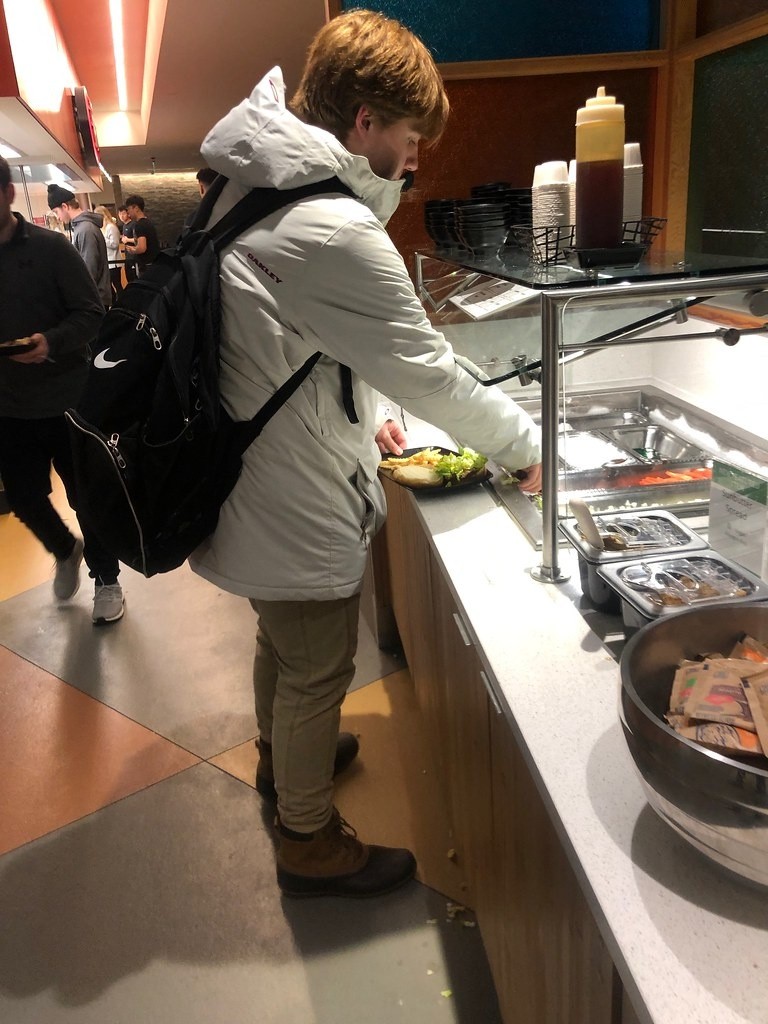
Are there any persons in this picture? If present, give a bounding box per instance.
[1,156,130,626]
[181,167,221,237]
[43,180,159,317]
[191,4,543,895]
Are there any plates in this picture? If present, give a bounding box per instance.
[369,446,494,497]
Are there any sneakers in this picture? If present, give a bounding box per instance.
[92,575,125,625]
[53,539,84,600]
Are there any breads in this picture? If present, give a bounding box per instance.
[392,465,444,488]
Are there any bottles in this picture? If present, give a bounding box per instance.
[575,86,625,248]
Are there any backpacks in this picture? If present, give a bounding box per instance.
[52,177,363,579]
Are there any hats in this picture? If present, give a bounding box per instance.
[47,184,75,210]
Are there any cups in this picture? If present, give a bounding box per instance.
[623,143,643,222]
[531,159,576,263]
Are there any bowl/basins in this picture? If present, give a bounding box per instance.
[424,182,531,256]
[617,602,768,886]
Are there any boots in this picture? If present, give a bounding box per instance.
[255,731,360,797]
[274,809,417,900]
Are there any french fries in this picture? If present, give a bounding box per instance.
[380,447,444,468]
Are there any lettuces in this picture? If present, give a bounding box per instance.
[435,446,489,487]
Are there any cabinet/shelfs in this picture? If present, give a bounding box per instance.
[379,472,639,1024]
